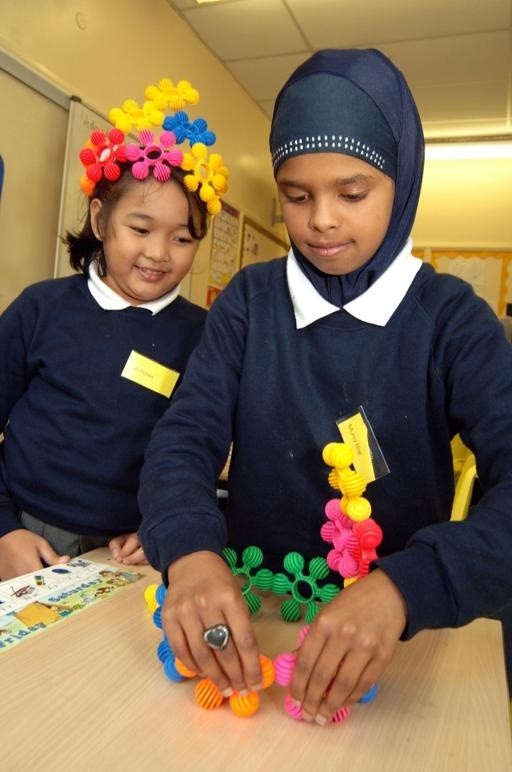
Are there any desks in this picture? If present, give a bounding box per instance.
[0,541,512,772]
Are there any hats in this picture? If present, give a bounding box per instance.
[271,73,397,179]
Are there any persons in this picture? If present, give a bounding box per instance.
[0,147,209,589]
[135,48,512,725]
